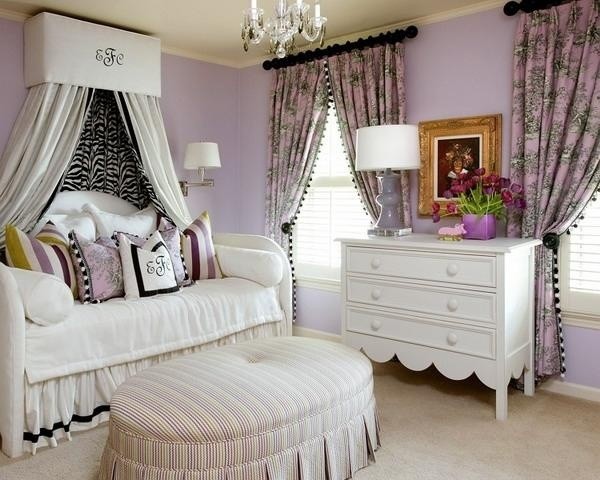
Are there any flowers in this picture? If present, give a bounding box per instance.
[430,163,530,226]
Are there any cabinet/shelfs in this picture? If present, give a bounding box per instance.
[338,235,542,424]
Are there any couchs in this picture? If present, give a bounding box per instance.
[1,189,293,461]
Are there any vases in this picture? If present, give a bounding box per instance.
[463,211,497,241]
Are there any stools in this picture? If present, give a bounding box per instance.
[97,334,382,480]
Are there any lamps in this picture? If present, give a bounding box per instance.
[238,1,329,60]
[174,136,222,198]
[354,123,423,237]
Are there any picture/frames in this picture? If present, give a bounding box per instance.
[419,113,504,219]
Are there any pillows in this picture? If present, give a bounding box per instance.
[210,242,285,287]
[1,265,77,326]
[3,205,226,303]
